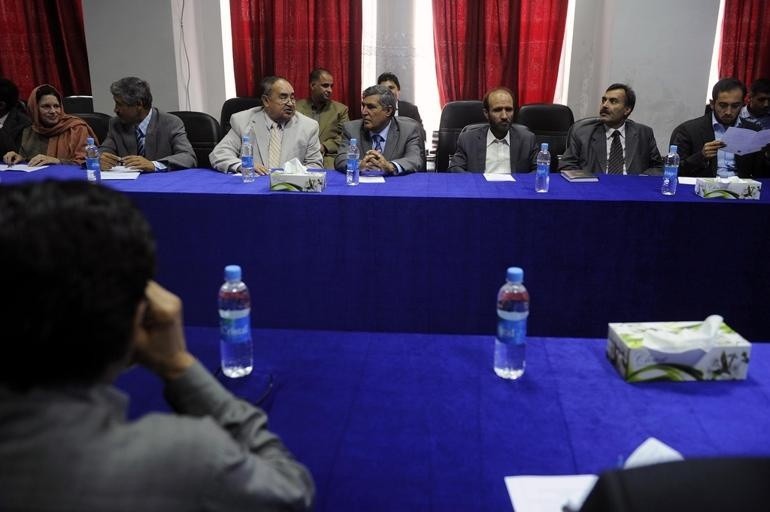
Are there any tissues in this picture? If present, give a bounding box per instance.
[606,314,753,384]
[694,175,762,201]
[269,156,326,192]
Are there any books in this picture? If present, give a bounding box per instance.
[561,170,599,183]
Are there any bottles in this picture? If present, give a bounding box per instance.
[492,267,530,380]
[535,143,551,193]
[346,138,360,186]
[86,138,101,185]
[661,145,681,196]
[217,264,253,378]
[241,136,255,183]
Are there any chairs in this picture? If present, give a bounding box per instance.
[166,112,220,168]
[19,95,111,145]
[435,101,489,172]
[220,97,264,140]
[516,104,574,173]
[565,117,634,150]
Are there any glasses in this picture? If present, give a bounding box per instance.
[269,97,295,105]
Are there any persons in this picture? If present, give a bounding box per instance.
[0,176,314,512]
[738,78,770,129]
[668,78,770,180]
[557,83,667,177]
[2,84,101,167]
[296,67,350,170]
[208,75,324,176]
[576,453,770,512]
[334,84,426,176]
[448,87,541,173]
[97,77,198,172]
[0,78,32,163]
[377,72,423,128]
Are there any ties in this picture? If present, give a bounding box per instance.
[373,135,382,150]
[135,126,144,156]
[268,124,282,168]
[608,130,623,175]
